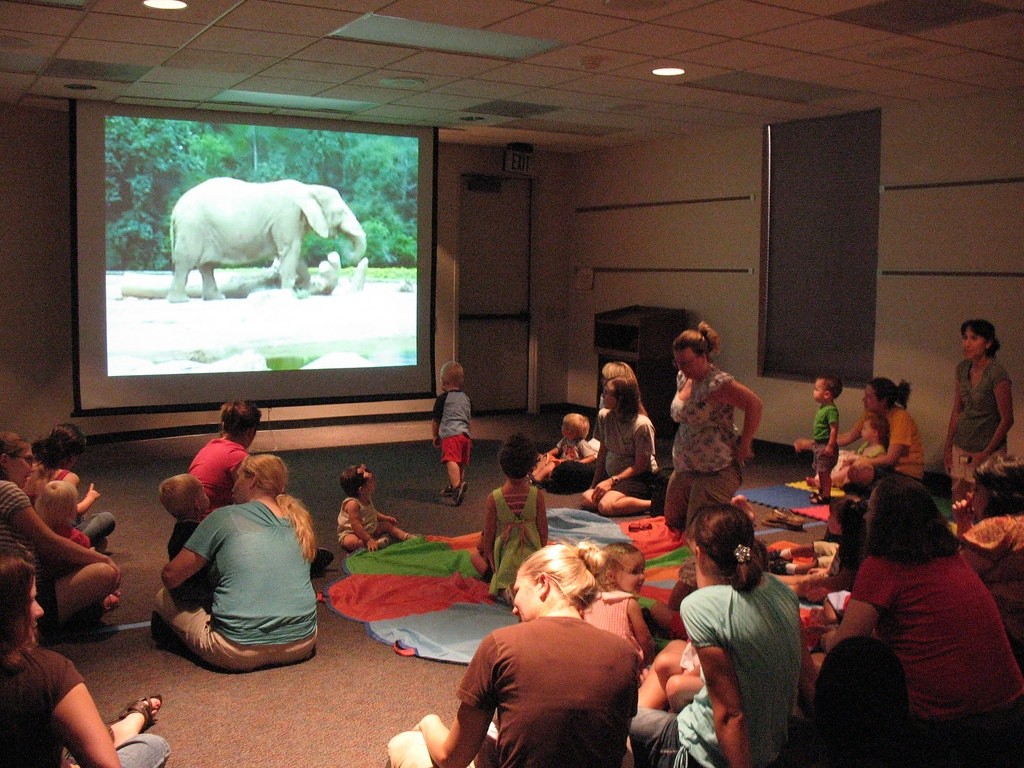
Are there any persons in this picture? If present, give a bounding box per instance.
[0,554,169,768]
[36,480,91,573]
[189,401,334,569]
[160,474,215,612]
[152,454,318,672]
[23,423,115,554]
[336,464,415,552]
[389,321,1024,768]
[0,432,121,639]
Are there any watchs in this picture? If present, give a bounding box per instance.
[612,475,619,485]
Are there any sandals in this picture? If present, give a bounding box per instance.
[440,484,454,497]
[104,693,162,741]
[454,481,468,506]
[809,490,831,506]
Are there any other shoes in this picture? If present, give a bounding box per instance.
[313,549,333,573]
[101,593,118,609]
[770,561,789,574]
[405,533,421,541]
[767,550,795,562]
[376,539,390,548]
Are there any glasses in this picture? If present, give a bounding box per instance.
[671,355,701,368]
[8,451,33,465]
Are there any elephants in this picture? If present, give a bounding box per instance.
[167,176,367,303]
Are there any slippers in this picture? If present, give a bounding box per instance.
[762,510,803,532]
